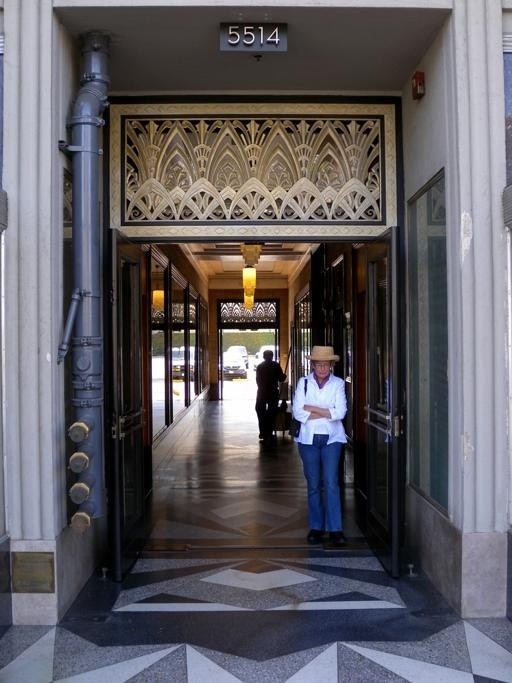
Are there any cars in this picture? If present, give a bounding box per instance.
[221,350,245,378]
[253,344,278,368]
[227,345,249,368]
[169,346,195,381]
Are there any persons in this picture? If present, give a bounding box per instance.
[289,344,352,548]
[255,349,287,442]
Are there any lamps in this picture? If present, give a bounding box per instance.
[151,263,164,311]
[239,263,257,310]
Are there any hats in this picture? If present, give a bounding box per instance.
[306,346,341,363]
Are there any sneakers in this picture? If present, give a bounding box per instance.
[306,529,345,549]
